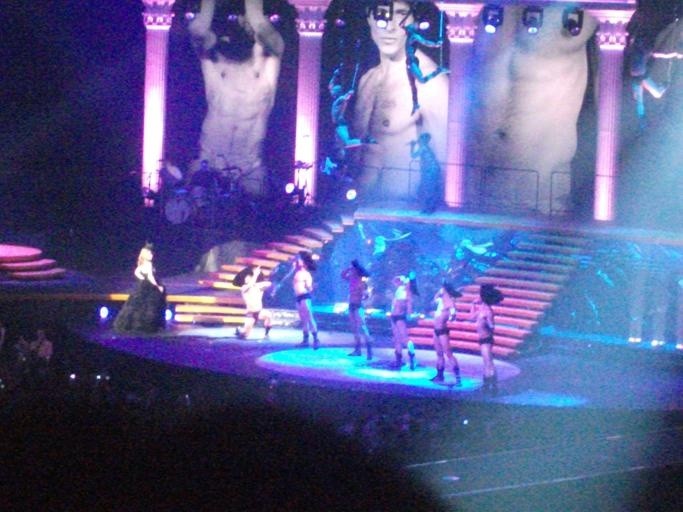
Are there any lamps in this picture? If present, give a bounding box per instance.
[481,3,504,35]
[407,0,433,30]
[370,1,395,29]
[562,5,585,37]
[521,3,543,36]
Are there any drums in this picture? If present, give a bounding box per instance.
[160,163,182,187]
[207,188,224,203]
[191,186,210,209]
[164,189,198,226]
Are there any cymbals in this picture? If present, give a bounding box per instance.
[229,172,236,174]
[223,166,236,171]
[223,176,231,179]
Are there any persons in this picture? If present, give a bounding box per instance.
[293,251,321,349]
[353,1,450,209]
[327,59,379,162]
[340,258,373,360]
[106,148,356,274]
[397,6,452,118]
[540,244,683,344]
[113,243,169,336]
[352,219,517,317]
[430,282,464,387]
[470,0,600,216]
[470,283,505,393]
[2,315,448,511]
[408,132,444,217]
[231,264,273,341]
[626,13,680,137]
[186,0,285,197]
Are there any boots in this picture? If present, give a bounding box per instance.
[453,365,461,387]
[234,326,241,336]
[312,332,319,349]
[348,344,361,356]
[296,331,308,347]
[489,372,497,396]
[432,364,444,382]
[408,350,415,369]
[263,326,271,340]
[477,374,489,392]
[388,351,401,366]
[367,343,373,359]
